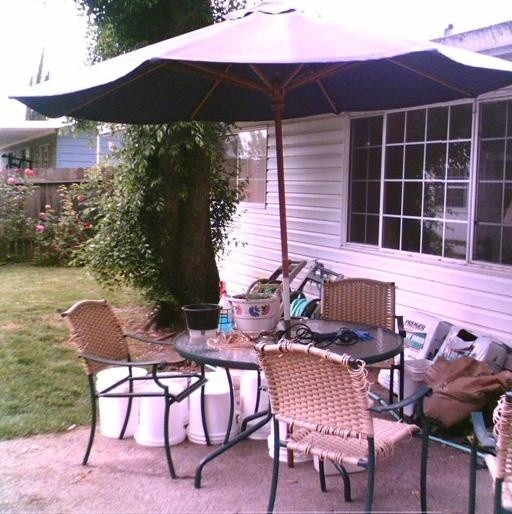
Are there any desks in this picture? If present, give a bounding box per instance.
[174,320,406,489]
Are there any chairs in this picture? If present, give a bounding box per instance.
[255,278,512,513]
[62,297,210,480]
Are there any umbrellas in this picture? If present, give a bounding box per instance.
[7,2,512,341]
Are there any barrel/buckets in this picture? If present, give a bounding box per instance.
[230,293,277,333]
[93,367,366,476]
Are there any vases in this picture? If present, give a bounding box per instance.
[180,304,224,341]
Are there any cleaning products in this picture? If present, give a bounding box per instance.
[217,293,235,332]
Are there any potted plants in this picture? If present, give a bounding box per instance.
[230,284,280,338]
